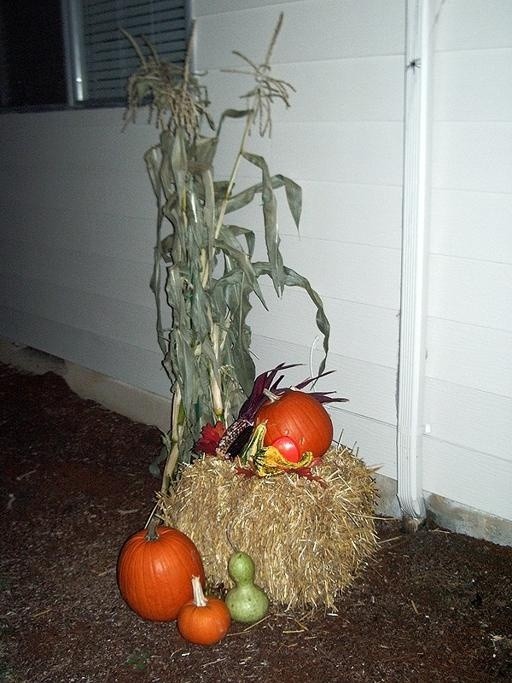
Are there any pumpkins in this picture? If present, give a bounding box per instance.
[117,516,206,623]
[240,388,333,478]
[178,575,229,645]
